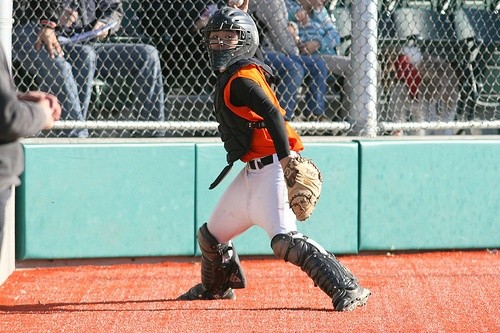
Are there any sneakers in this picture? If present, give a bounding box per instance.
[175,284,236,300]
[336,288,372,311]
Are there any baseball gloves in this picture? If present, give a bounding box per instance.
[282,156,324,221]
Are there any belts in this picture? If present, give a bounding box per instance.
[247,155,276,170]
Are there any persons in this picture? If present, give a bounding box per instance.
[174,7,372,314]
[194,0,341,123]
[12,0,166,139]
[0,42,65,290]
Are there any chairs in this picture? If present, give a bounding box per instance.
[327,0,500,107]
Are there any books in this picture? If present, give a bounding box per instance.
[59,20,118,46]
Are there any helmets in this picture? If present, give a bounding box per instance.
[200,7,260,72]
